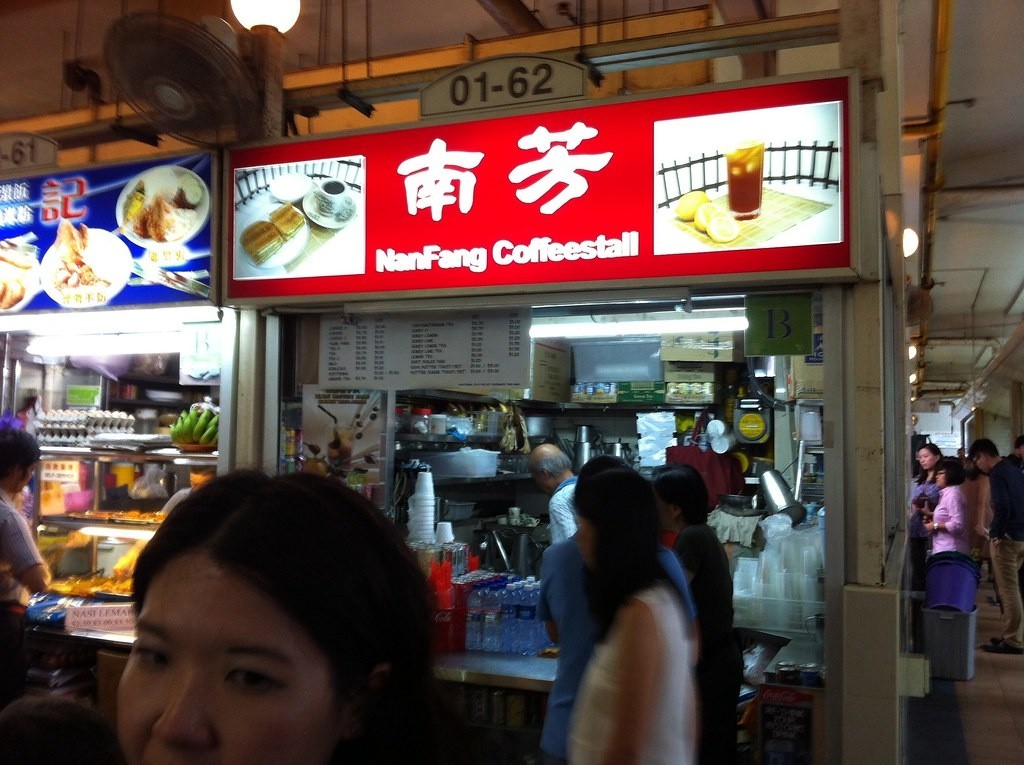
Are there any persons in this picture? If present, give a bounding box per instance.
[969,438,1024,654]
[117,469,433,765]
[529,444,581,544]
[0,427,53,713]
[537,454,632,765]
[652,464,743,765]
[923,459,968,555]
[567,466,696,765]
[907,443,943,653]
[957,435,1024,616]
[160,465,217,514]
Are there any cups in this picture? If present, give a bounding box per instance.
[436,522,454,544]
[510,518,521,526]
[727,140,764,221]
[509,507,521,518]
[804,613,825,643]
[414,472,435,542]
[327,424,354,478]
[733,532,824,613]
[497,517,507,525]
[317,179,354,217]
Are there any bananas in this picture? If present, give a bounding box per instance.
[169,409,219,447]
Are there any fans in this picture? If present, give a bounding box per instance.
[907,275,933,327]
[104,12,264,149]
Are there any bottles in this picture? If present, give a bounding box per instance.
[464,576,556,654]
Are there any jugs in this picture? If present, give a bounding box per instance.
[522,516,540,527]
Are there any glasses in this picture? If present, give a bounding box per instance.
[973,455,981,465]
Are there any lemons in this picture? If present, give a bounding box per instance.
[675,190,740,242]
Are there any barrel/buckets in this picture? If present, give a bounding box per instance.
[923,551,982,613]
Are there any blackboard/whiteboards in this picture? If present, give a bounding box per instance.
[756,700,828,765]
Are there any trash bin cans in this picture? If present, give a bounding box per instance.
[919,550,983,681]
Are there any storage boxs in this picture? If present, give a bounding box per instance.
[789,324,823,398]
[410,449,501,478]
[97,647,131,735]
[443,501,476,521]
[495,330,744,406]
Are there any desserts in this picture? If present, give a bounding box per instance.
[239,202,305,266]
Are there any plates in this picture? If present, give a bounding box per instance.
[240,203,309,269]
[41,229,132,308]
[146,390,184,402]
[303,190,356,229]
[115,165,210,249]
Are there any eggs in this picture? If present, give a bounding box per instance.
[44,409,127,428]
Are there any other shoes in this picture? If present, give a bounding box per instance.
[984,637,1024,655]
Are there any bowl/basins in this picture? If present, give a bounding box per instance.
[718,494,760,517]
[271,173,311,205]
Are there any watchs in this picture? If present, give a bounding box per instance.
[934,522,939,532]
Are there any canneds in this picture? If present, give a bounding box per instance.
[390,542,501,611]
[572,382,618,395]
[667,382,714,395]
[393,407,447,435]
[280,427,304,475]
[774,661,822,689]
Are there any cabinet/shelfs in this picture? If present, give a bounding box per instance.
[60,372,195,412]
[32,447,218,600]
[369,390,547,531]
